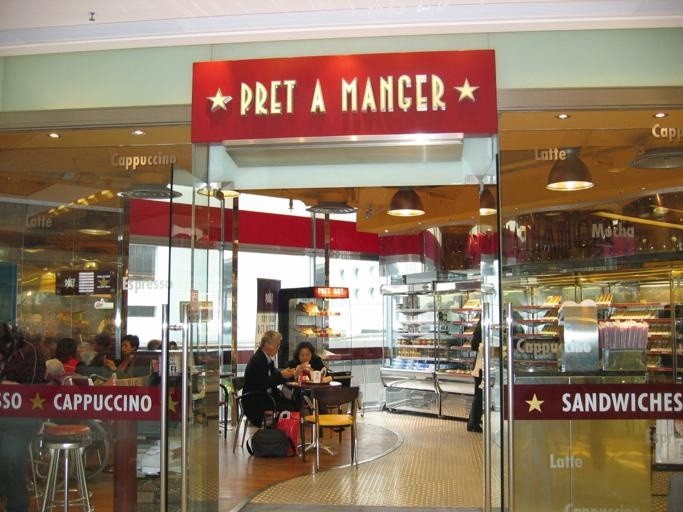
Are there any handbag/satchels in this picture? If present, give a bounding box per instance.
[246,428,296,457]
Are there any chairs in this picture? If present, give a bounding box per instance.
[26,370,358,510]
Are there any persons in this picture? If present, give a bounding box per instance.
[147,340,177,349]
[45,335,139,383]
[0,321,46,512]
[467,313,483,432]
[148,359,160,386]
[241,331,326,429]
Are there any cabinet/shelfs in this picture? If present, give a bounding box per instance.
[276,286,349,370]
[379,250,683,423]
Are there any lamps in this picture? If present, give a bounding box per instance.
[544,149,594,191]
[479,184,496,215]
[385,188,425,218]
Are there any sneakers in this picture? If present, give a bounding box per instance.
[467,423,482,432]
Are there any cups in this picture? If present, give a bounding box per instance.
[311,370,322,383]
